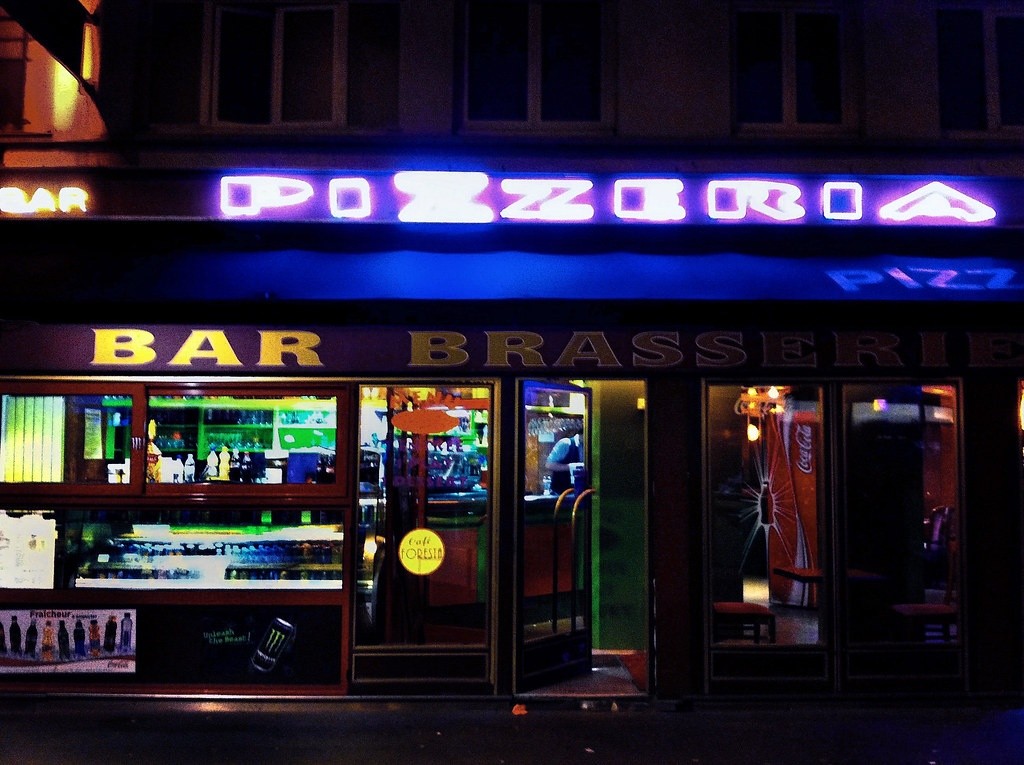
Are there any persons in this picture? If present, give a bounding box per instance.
[545,429,585,496]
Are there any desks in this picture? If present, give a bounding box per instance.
[773,567,880,646]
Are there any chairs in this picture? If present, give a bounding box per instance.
[714,602,777,643]
[892,541,960,645]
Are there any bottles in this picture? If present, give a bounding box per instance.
[185,454,195,483]
[120,613,132,652]
[207,446,218,476]
[172,454,184,484]
[73,621,86,656]
[241,451,254,484]
[58,620,70,659]
[88,620,101,656]
[42,621,54,661]
[9,616,22,656]
[761,480,774,525]
[104,616,117,652]
[230,449,241,482]
[108,543,342,580]
[219,447,231,479]
[24,619,38,658]
[0,622,7,654]
